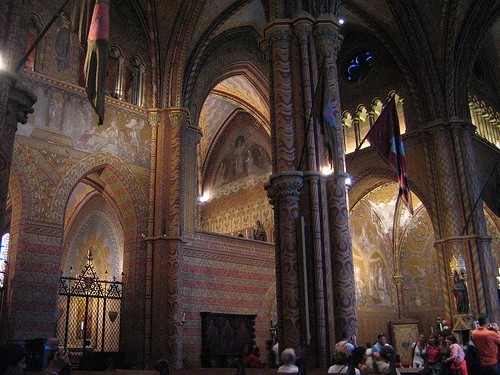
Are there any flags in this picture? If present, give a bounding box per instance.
[310,55,342,187]
[480,158,500,219]
[83,0,110,126]
[367,94,415,215]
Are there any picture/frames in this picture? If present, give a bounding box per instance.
[388,317,422,362]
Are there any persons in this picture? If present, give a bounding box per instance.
[203,315,252,357]
[411,312,500,375]
[252,221,266,241]
[469,313,500,375]
[0,337,71,375]
[271,330,398,374]
[452,269,469,313]
